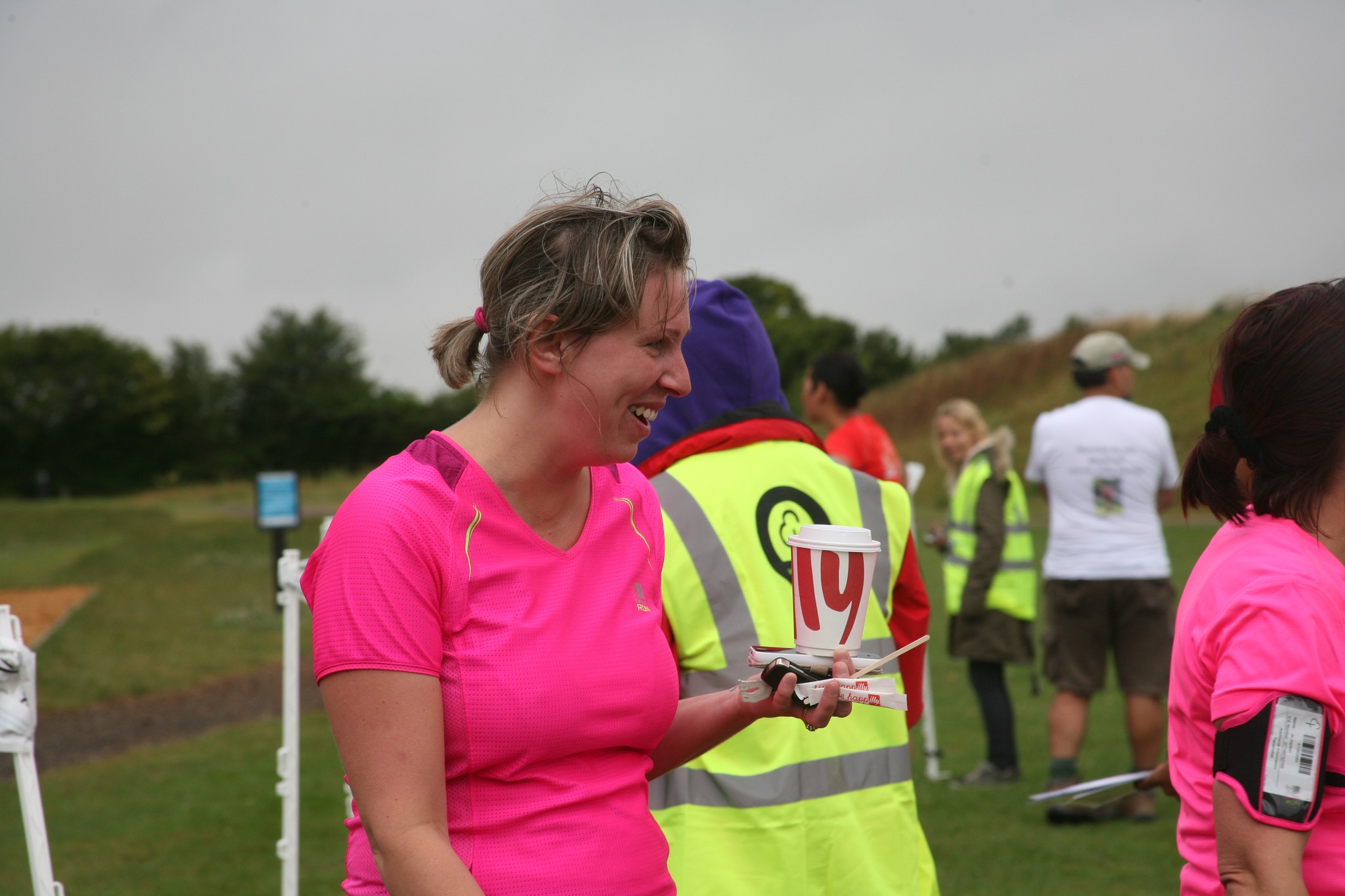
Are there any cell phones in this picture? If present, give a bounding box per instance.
[749,645,885,670]
[1260,695,1325,823]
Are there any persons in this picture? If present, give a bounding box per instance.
[801,350,909,488]
[1167,277,1345,896]
[927,398,1038,790]
[1027,330,1181,819]
[301,173,855,896]
[630,280,942,896]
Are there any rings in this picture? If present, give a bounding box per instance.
[803,708,818,731]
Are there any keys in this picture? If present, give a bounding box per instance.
[761,656,825,706]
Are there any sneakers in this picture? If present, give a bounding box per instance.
[949,761,1019,787]
[1042,774,1158,821]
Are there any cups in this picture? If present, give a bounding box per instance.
[787,525,882,656]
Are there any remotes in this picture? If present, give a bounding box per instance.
[760,657,825,702]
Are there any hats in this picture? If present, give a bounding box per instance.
[1069,332,1151,371]
